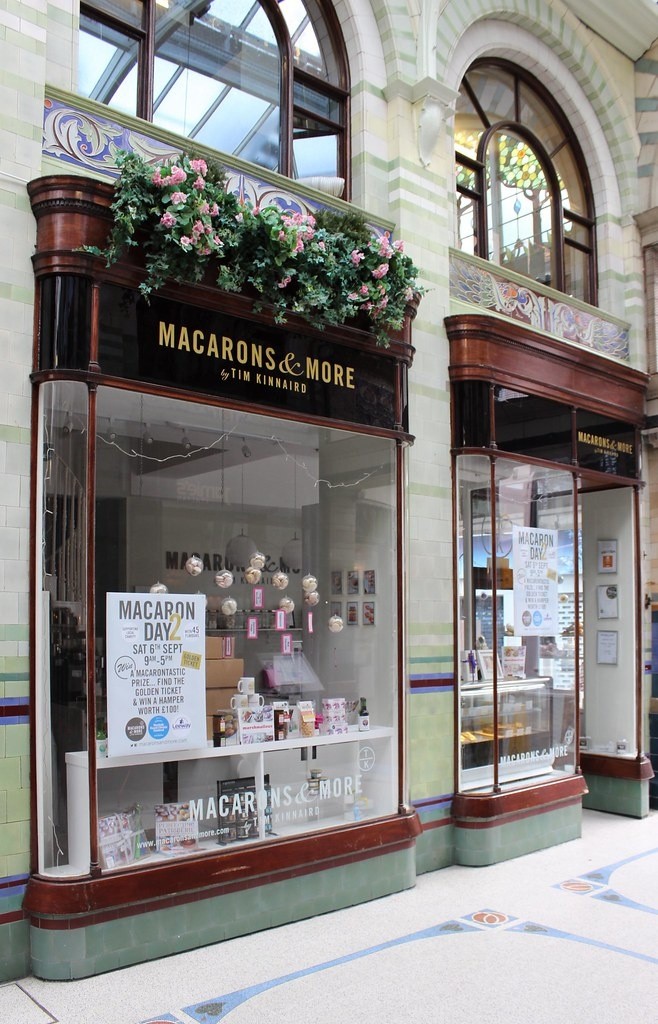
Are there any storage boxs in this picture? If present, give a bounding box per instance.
[205,634,245,740]
[220,706,275,747]
[97,808,150,869]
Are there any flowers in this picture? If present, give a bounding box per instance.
[69,146,432,350]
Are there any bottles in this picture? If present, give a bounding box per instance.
[358,698,370,731]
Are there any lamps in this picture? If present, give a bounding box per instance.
[141,423,155,445]
[237,438,252,458]
[181,430,193,449]
[61,411,74,433]
[107,417,117,441]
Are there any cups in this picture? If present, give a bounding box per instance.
[230,676,265,709]
